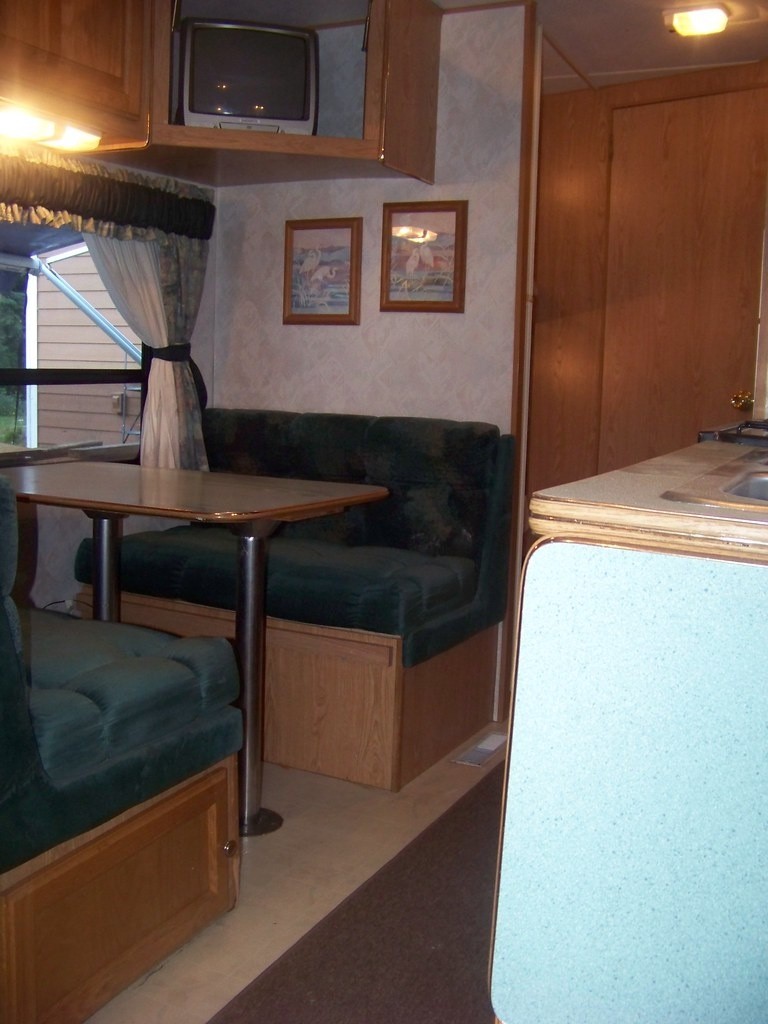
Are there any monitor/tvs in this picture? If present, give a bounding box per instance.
[167,17,321,136]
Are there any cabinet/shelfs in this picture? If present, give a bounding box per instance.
[0,0,441,189]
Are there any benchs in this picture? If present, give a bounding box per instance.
[0,473,242,1024]
[73,408,511,793]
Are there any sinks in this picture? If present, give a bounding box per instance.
[660,449,768,513]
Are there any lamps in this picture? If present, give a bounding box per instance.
[661,1,730,38]
[1,107,105,155]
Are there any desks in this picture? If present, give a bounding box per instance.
[1,460,389,836]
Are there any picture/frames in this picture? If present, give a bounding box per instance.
[282,217,362,324]
[379,199,468,314]
